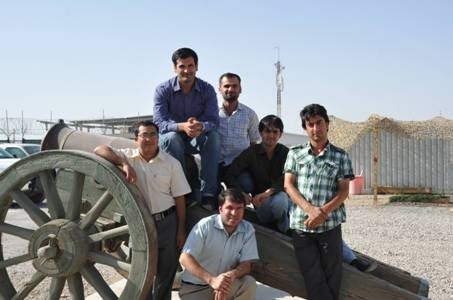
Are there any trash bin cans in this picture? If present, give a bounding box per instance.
[349,175,364,195]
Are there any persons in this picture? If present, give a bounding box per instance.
[95,121,191,300]
[178,187,261,300]
[152,48,221,213]
[224,115,378,273]
[218,73,260,194]
[283,104,355,300]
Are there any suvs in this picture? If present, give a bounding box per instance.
[2,141,40,160]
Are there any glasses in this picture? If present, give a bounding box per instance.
[138,133,158,140]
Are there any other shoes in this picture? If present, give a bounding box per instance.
[186,197,198,209]
[353,258,377,272]
[200,197,218,212]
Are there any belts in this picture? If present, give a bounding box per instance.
[181,280,209,286]
[150,206,176,221]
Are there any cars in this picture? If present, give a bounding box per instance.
[0,144,21,175]
